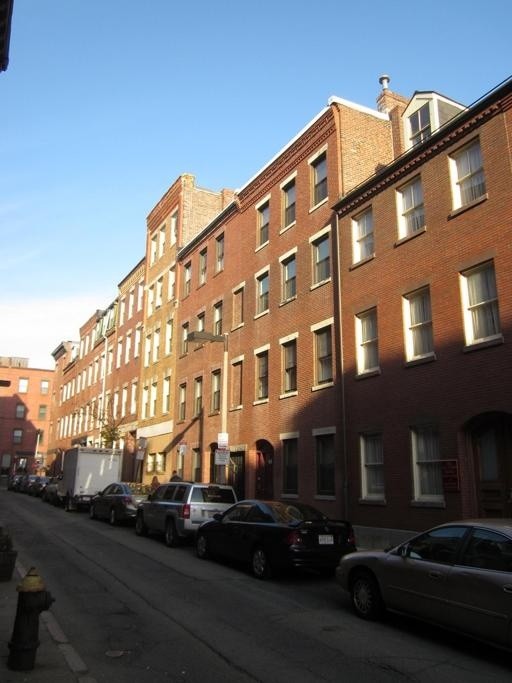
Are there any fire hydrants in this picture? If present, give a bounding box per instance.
[8,566,56,671]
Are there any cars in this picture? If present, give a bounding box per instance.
[330,518,512,657]
[7,472,59,506]
[194,499,357,579]
[88,481,157,526]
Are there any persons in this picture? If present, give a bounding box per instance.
[169,470,182,482]
[152,476,159,492]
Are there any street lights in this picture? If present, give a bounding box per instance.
[183,330,228,484]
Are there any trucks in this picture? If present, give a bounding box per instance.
[57,445,124,512]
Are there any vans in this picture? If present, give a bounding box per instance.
[135,480,238,547]
